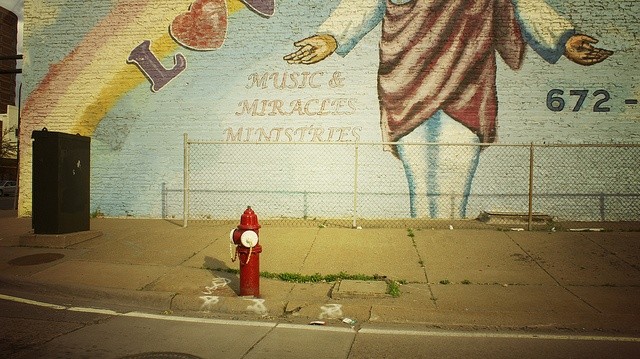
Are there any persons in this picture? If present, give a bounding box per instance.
[282,0,616,222]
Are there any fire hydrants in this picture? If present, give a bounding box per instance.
[228,206,263,297]
